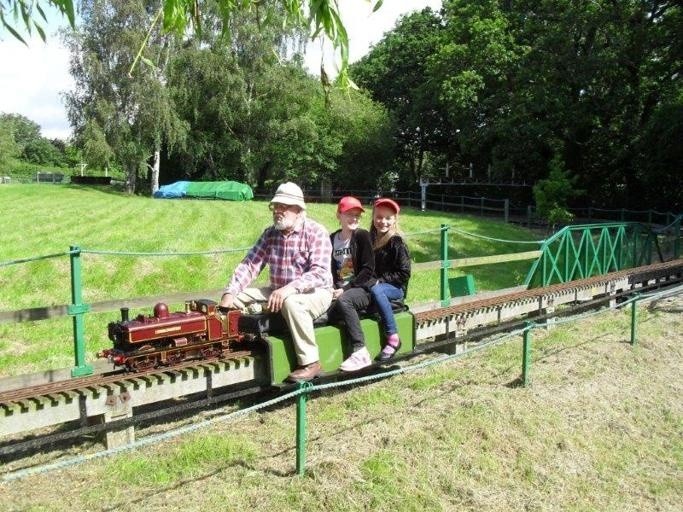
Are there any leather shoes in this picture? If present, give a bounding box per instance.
[378,338,401,360]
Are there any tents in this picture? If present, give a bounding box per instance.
[153,180,255,202]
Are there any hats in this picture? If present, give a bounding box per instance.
[338,197,365,214]
[374,198,400,214]
[269,181,308,210]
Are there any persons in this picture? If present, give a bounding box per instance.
[368,198,411,362]
[220,182,334,383]
[329,196,376,372]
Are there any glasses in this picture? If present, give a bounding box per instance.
[268,203,295,213]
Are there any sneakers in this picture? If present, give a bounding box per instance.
[287,362,321,382]
[338,351,373,372]
